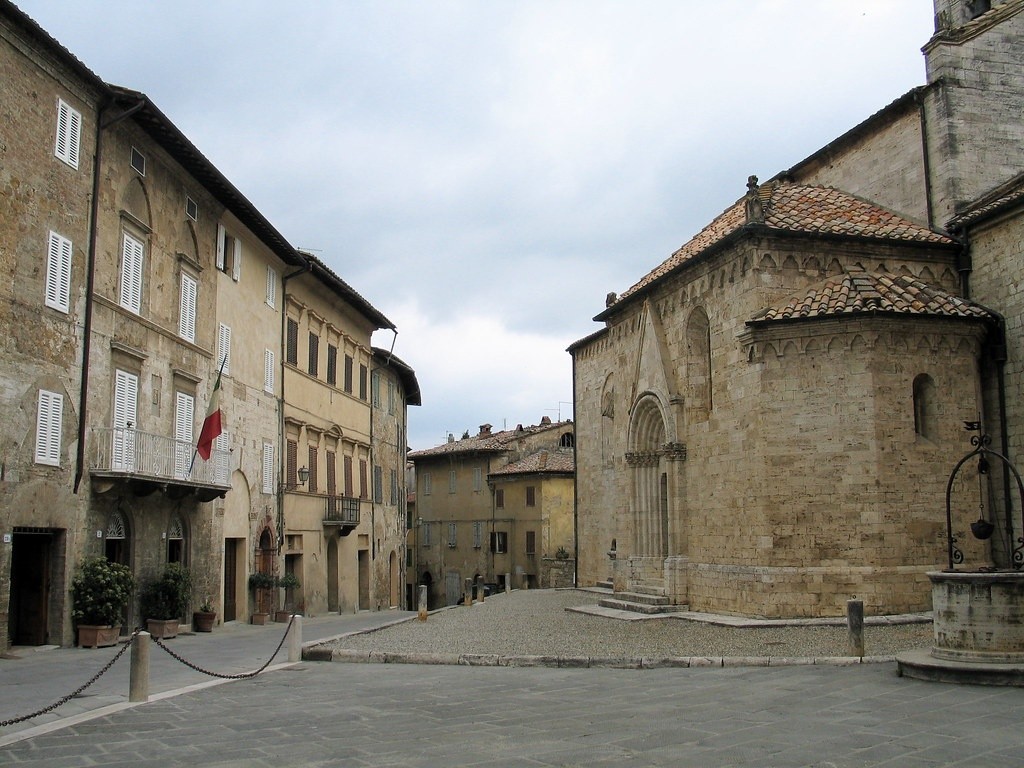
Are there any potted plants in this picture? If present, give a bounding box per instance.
[249,572,279,626]
[142,561,194,638]
[193,599,216,632]
[68,554,139,650]
[275,572,302,623]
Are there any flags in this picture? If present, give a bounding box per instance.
[197,370,223,462]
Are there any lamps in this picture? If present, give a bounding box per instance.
[276,465,310,500]
